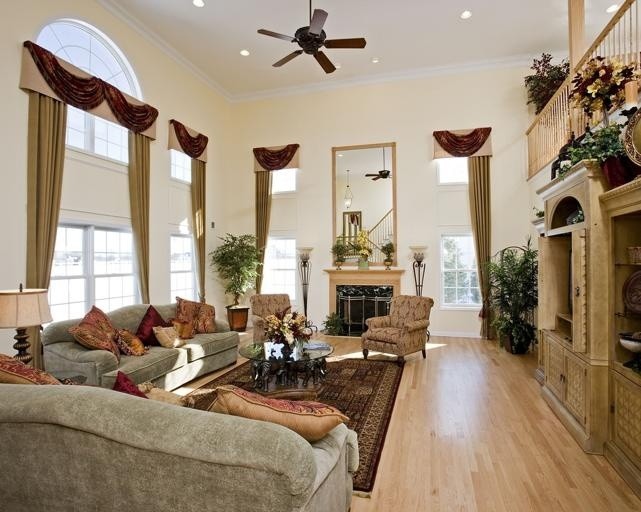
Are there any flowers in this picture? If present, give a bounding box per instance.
[263,307,320,343]
[566,56,637,155]
[356,229,373,258]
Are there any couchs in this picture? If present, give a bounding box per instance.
[40,302,241,394]
[0,383,360,512]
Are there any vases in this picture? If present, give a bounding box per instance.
[280,339,294,362]
[356,257,369,272]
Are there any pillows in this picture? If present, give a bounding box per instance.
[68,296,215,356]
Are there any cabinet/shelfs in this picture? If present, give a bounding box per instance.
[323,268,406,339]
[533,158,641,510]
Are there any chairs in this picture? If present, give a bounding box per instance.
[358,294,436,367]
[249,292,293,344]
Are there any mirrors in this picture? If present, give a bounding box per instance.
[332,141,396,267]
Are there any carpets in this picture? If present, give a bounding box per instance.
[177,353,407,493]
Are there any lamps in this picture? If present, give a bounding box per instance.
[0,281,54,367]
[295,246,318,334]
[343,170,353,210]
[408,245,430,342]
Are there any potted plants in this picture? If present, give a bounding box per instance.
[379,241,395,271]
[481,234,539,355]
[207,230,269,332]
[329,238,351,270]
[590,121,637,189]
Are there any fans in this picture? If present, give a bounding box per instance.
[364,147,393,181]
[255,1,368,75]
